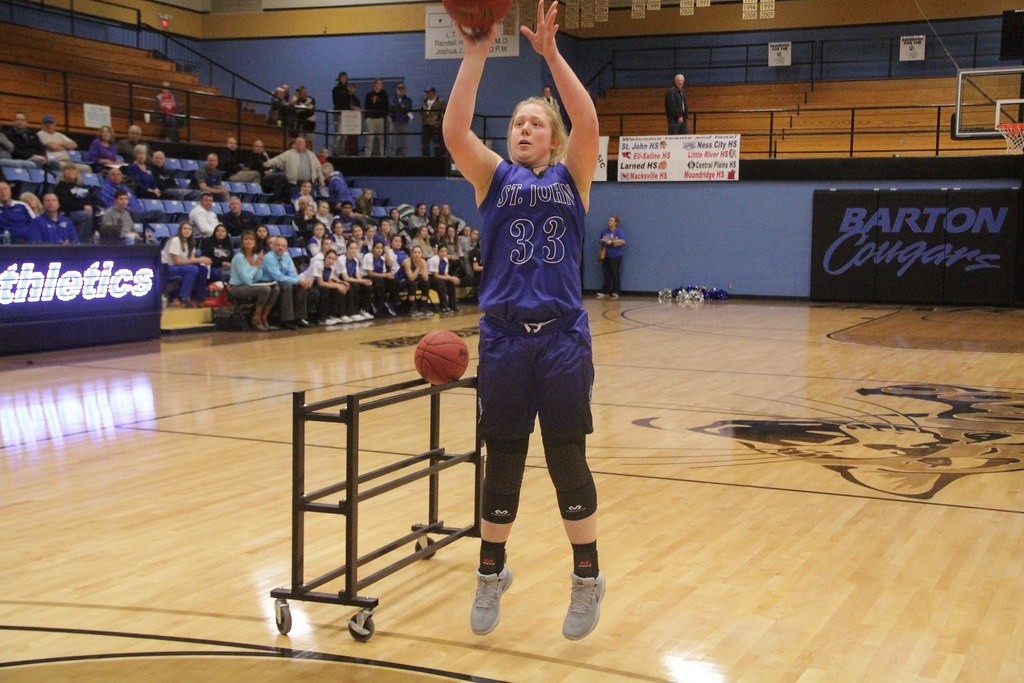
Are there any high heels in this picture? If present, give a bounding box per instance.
[263,320,276,330]
[252,319,268,331]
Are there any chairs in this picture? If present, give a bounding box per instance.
[0,148,450,332]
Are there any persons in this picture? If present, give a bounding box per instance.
[543,86,559,112]
[1,70,484,333]
[442,0,605,640]
[664,73,689,135]
[596,214,626,297]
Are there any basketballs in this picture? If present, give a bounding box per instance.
[413,328,470,386]
[440,0,515,28]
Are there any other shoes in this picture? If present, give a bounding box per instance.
[597,291,610,296]
[612,291,618,296]
[283,302,463,330]
[177,297,192,308]
[193,298,203,306]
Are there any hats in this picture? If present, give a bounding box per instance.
[425,86,436,93]
[43,116,55,124]
[398,84,405,89]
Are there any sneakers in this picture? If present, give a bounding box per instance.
[562,573,606,640]
[470,564,513,635]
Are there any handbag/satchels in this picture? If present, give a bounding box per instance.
[597,246,606,262]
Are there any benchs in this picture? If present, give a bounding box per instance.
[594,73,1024,159]
[0,20,316,151]
